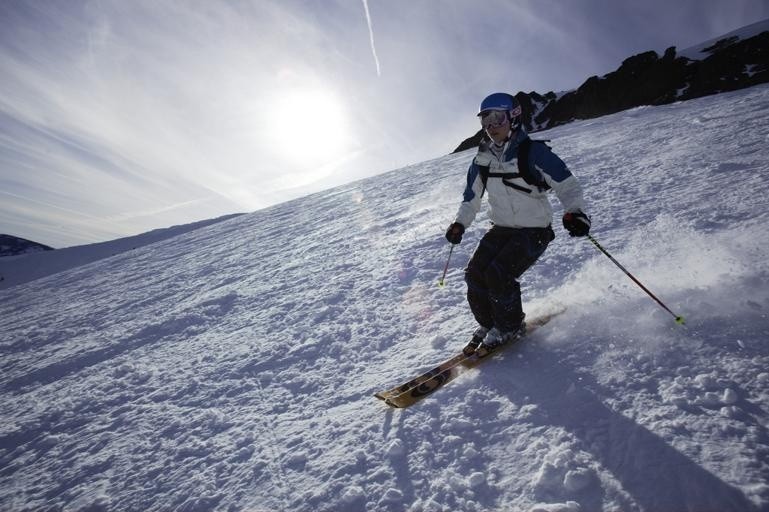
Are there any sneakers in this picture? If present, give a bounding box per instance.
[471,319,527,348]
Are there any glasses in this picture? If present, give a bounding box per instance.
[482,111,508,129]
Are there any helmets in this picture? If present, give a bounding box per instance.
[477,92,523,125]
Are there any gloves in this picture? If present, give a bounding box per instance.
[561,210,593,238]
[445,221,466,245]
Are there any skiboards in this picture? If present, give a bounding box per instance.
[374,309,565,408]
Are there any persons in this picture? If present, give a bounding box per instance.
[445,93,590,358]
